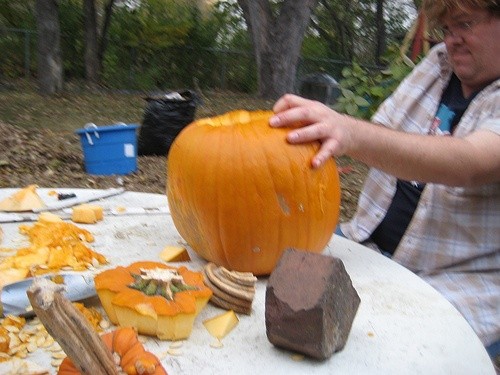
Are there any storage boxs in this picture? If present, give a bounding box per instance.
[73,123,142,175]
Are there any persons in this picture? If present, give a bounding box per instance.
[269,0,500,347]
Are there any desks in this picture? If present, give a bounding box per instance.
[0,187,496,375]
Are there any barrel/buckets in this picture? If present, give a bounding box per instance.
[75,122,141,176]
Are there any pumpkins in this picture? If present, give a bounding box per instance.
[165,109,342,277]
[26,278,169,375]
[94,260,214,341]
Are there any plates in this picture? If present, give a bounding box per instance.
[1,272,97,316]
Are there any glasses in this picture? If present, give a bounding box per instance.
[435,11,494,42]
